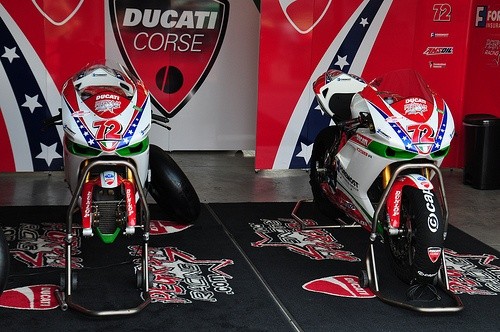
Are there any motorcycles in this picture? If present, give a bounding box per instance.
[46,63,172,275]
[309,68,458,288]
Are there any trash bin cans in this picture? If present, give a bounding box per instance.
[462,113,500,190]
